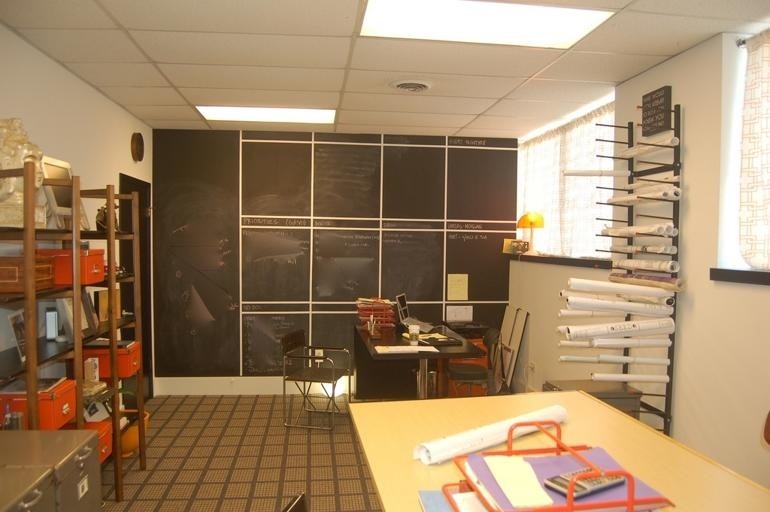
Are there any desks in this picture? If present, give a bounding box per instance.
[347,389,770,512]
[353,325,488,400]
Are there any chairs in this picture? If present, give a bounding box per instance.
[444,324,499,396]
[282,491,307,512]
[281,328,351,431]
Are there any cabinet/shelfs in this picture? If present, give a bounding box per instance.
[543,379,642,420]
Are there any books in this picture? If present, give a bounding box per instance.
[416,447,669,512]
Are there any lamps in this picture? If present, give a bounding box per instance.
[518,212,545,256]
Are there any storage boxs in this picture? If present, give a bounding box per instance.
[0,161,143,512]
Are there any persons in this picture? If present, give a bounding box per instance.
[0,120,51,229]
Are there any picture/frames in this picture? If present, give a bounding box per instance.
[511,241,529,257]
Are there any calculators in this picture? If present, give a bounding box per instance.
[544,468,625,498]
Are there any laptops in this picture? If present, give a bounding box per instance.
[396,292,434,333]
